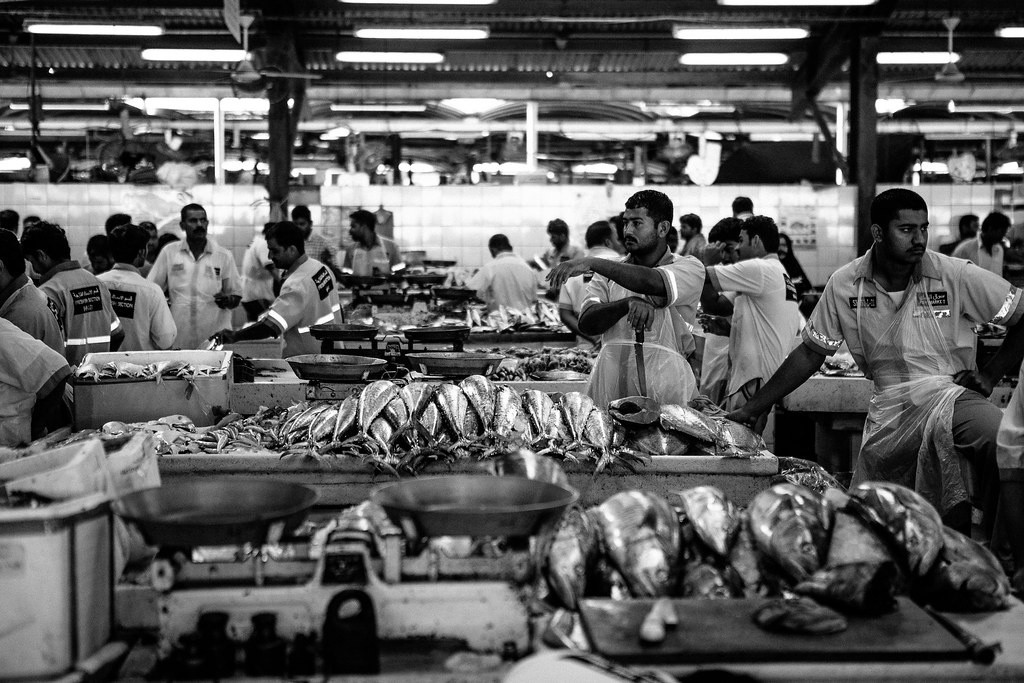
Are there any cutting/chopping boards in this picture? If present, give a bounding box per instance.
[575,594,969,663]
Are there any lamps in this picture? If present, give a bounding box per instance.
[671,23,812,41]
[352,24,491,41]
[23,19,165,37]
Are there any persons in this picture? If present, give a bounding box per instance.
[461,188,1024,583]
[0,203,406,447]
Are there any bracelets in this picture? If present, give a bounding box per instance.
[232,330,238,342]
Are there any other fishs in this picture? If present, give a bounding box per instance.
[821,354,867,378]
[403,266,480,289]
[545,479,1011,608]
[347,300,573,334]
[58,345,769,477]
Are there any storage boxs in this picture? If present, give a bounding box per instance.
[72,350,235,430]
[0,438,107,506]
[0,506,114,682]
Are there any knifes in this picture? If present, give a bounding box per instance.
[634,326,647,396]
